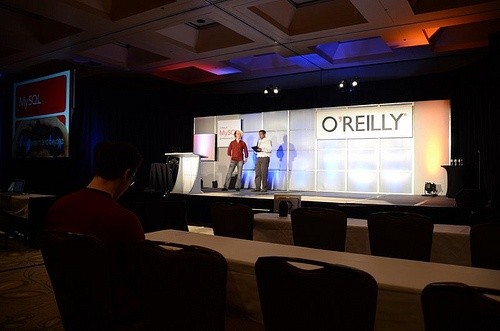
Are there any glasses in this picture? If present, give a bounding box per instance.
[121,169,137,185]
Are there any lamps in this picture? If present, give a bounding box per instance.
[351,75,360,88]
[338,79,347,88]
[274,83,280,94]
[264,84,273,94]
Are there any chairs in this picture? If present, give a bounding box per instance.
[255,257,378,331]
[211,202,255,240]
[469,224,500,270]
[146,201,189,234]
[421,283,500,331]
[367,211,434,262]
[292,207,347,251]
[118,241,228,331]
[37,231,122,331]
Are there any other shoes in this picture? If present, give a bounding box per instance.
[222,188,227,191]
[260,189,267,192]
[235,188,240,192]
[251,188,261,192]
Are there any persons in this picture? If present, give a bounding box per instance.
[45,138,144,243]
[220,130,248,191]
[252,130,272,192]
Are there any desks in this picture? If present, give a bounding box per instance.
[253,212,472,267]
[149,163,179,190]
[0,192,56,240]
[144,228,500,331]
